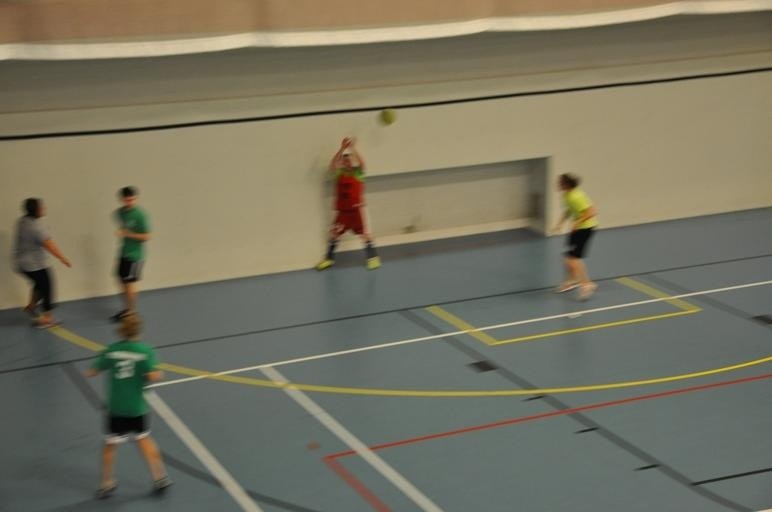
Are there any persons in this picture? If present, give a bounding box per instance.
[111,187,154,321]
[86,312,173,500]
[316,137,381,269]
[546,173,598,300]
[15,198,72,324]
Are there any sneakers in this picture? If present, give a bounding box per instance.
[154,477,174,492]
[317,260,335,270]
[367,256,381,270]
[108,308,137,324]
[24,304,63,329]
[553,280,597,301]
[94,483,118,500]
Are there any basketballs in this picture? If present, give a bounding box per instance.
[379,110,396,125]
